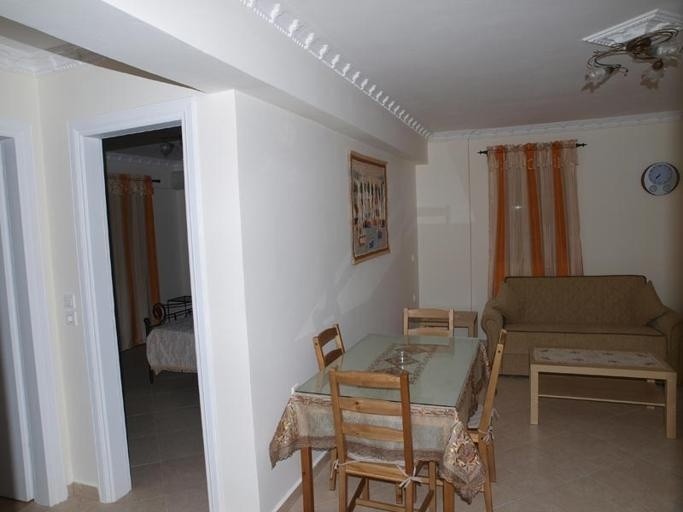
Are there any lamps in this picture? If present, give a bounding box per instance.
[578,9,681,98]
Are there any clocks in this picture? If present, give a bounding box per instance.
[641,161,680,198]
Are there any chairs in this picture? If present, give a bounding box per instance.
[464,330,507,512]
[312,323,348,492]
[328,366,436,511]
[403,307,454,337]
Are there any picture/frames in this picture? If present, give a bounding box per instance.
[349,149,391,265]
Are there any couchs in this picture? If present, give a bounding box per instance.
[480,275,682,385]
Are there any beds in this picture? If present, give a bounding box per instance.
[145,303,198,385]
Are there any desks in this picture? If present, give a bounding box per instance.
[528,346,677,440]
[267,332,500,511]
[418,311,478,338]
